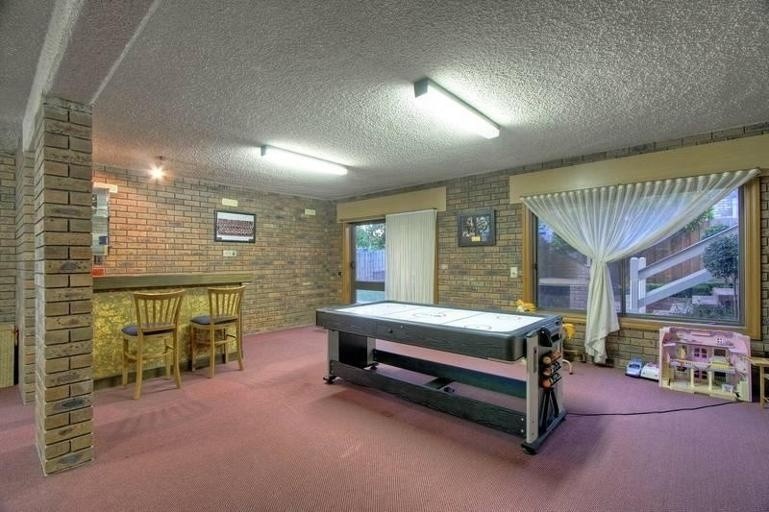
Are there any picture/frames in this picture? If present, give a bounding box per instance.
[214,210,256,244]
[457,209,496,246]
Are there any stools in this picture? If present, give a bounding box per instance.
[751,357,769,408]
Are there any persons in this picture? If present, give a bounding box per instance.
[217,218,254,235]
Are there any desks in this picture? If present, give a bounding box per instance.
[316,300,576,446]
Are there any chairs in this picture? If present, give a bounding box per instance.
[190,285,246,378]
[121,289,188,398]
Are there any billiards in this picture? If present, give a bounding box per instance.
[542,351,562,387]
